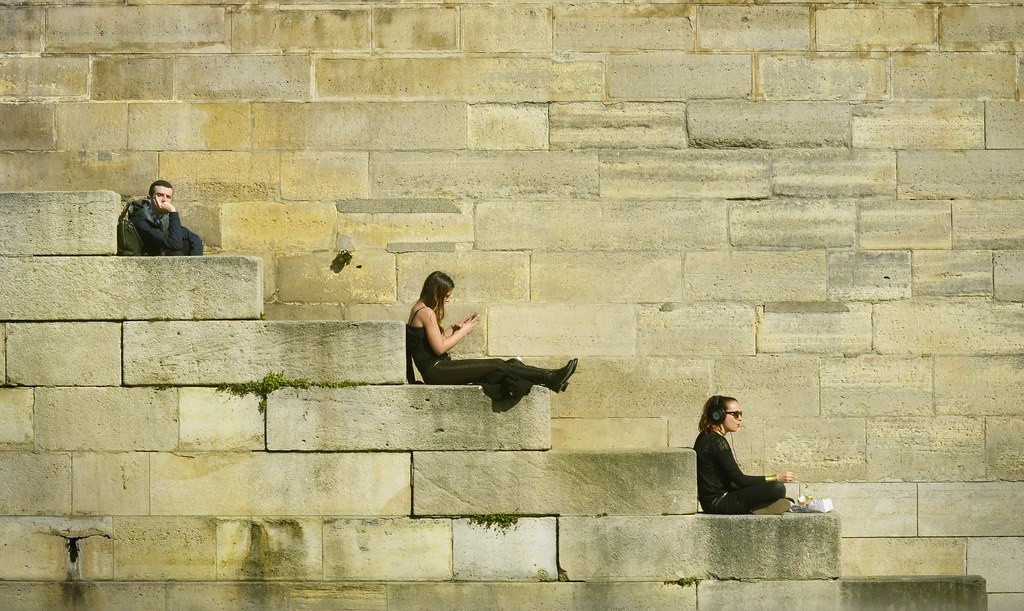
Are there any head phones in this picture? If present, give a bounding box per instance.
[709,395,726,424]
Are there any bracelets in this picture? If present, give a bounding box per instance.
[776,474,780,482]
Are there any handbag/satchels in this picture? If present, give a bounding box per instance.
[117,202,144,256]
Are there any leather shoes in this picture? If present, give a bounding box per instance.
[549,358,578,392]
[544,360,574,394]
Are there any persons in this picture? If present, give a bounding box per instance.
[692,395,795,514]
[406,270,579,403]
[117,179,203,256]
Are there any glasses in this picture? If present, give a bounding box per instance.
[726,411,742,419]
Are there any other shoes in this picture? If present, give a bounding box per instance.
[753,498,791,516]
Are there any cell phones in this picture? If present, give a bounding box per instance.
[471,312,477,321]
